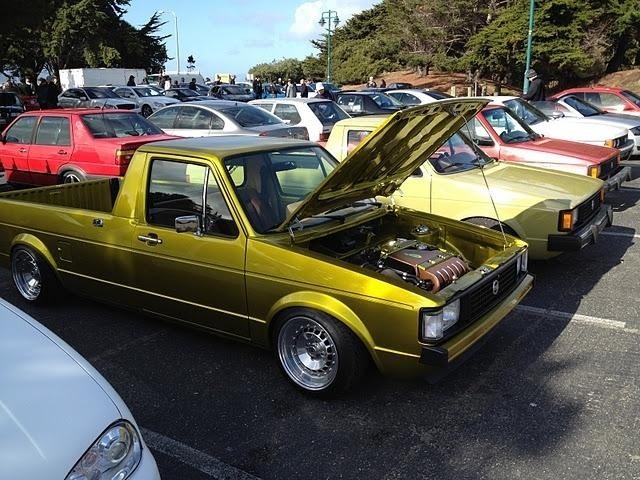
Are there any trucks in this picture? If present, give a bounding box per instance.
[148,73,205,85]
[58,68,153,90]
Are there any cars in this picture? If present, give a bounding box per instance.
[0,297,160,479]
[0,80,356,112]
[384,89,456,109]
[146,100,310,145]
[235,98,352,143]
[333,91,408,116]
[466,94,635,163]
[0,107,193,180]
[544,86,640,114]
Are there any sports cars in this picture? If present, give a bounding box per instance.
[542,95,640,157]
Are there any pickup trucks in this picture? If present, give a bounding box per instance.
[315,103,632,197]
[184,114,611,261]
[0,97,535,395]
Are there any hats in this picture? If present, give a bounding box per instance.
[525,68,537,78]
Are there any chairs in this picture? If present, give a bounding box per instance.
[238,189,273,233]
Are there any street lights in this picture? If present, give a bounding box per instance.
[317,11,340,82]
[158,11,180,75]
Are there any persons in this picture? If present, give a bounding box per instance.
[1,77,63,105]
[366,75,377,88]
[520,68,546,102]
[380,79,386,87]
[125,73,338,106]
